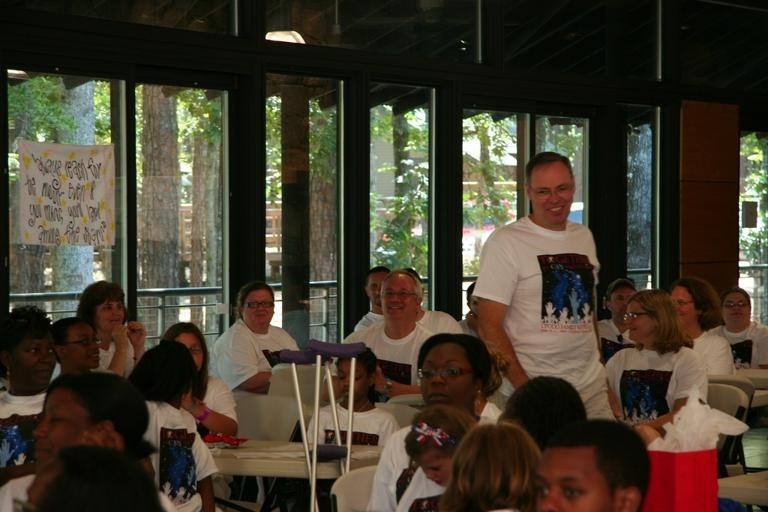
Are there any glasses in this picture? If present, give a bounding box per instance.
[723,300,748,309]
[382,289,417,299]
[68,337,102,346]
[247,301,274,308]
[622,311,649,321]
[417,367,472,381]
[529,183,572,200]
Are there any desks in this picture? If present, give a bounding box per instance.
[201,437,382,510]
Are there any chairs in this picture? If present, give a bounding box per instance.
[0,331,767,512]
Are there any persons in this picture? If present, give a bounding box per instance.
[2,281,238,512]
[477,149,624,427]
[210,281,300,394]
[294,266,478,511]
[597,279,767,445]
[367,332,650,512]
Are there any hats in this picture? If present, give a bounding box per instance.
[607,278,634,296]
